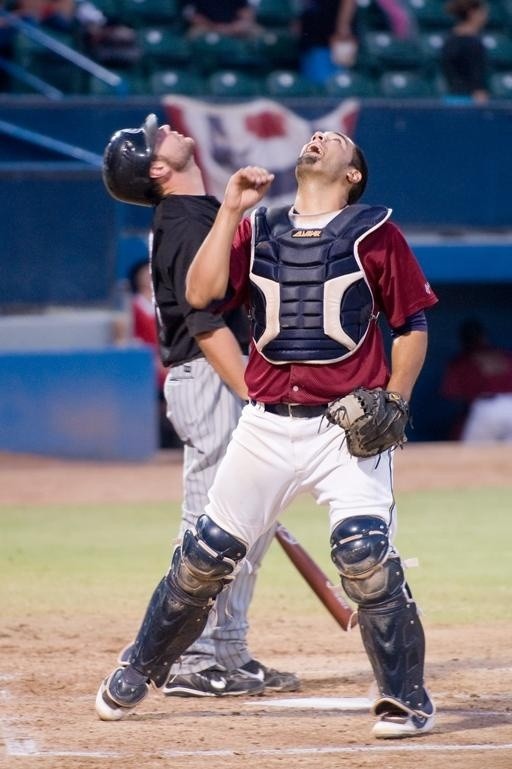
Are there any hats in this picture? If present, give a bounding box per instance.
[101,112,161,211]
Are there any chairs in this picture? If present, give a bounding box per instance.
[16,0,511,103]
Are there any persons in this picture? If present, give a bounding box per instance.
[96,131,437,738]
[102,112,298,697]
[117,260,183,447]
[440,320,512,441]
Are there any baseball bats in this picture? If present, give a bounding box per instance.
[270,520,359,631]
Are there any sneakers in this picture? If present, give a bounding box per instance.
[162,661,265,699]
[228,657,302,692]
[94,671,134,722]
[371,709,435,739]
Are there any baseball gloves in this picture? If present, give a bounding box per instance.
[325,386,413,458]
